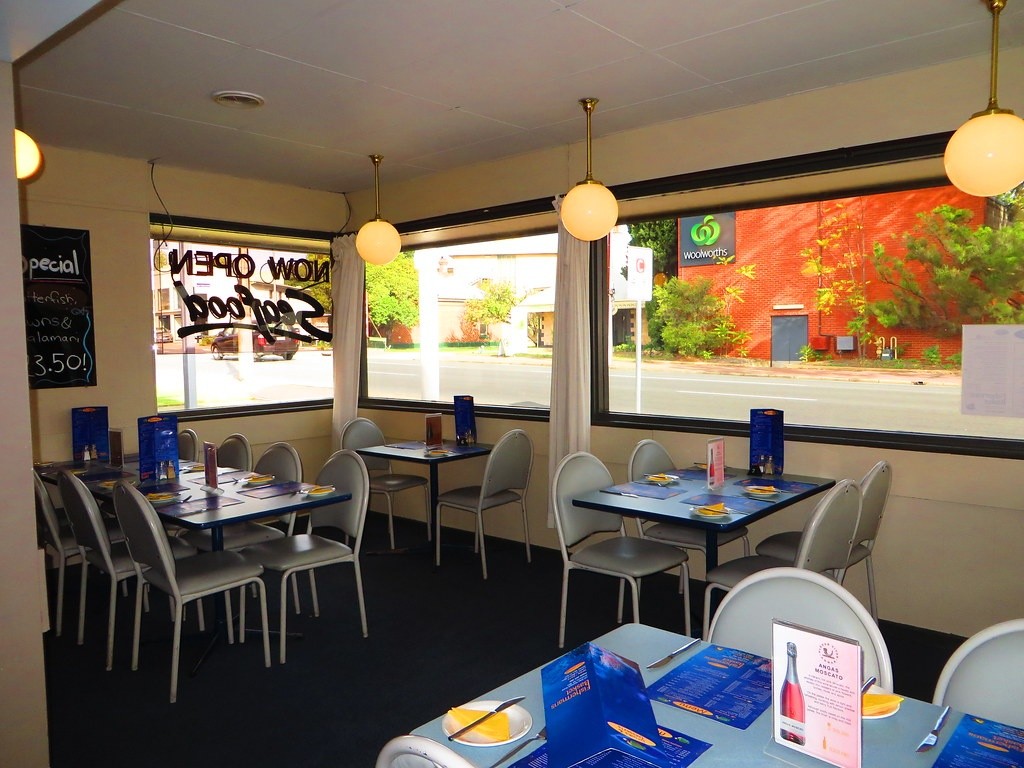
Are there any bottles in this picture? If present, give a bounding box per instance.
[710,448,715,485]
[779,642,807,747]
[82,443,98,461]
[751,455,773,475]
[159,459,176,480]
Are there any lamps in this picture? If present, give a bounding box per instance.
[356,154,401,265]
[15,129,42,179]
[943,0,1024,198]
[560,97,619,240]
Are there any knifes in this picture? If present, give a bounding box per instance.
[449,696,526,741]
[915,705,952,752]
[600,489,638,497]
[646,638,699,667]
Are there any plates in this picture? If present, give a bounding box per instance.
[57,466,88,474]
[689,505,732,519]
[241,474,275,485]
[441,700,534,748]
[646,474,679,484]
[425,450,451,457]
[183,463,205,471]
[300,485,336,497]
[861,685,901,719]
[697,462,727,470]
[96,479,135,490]
[146,492,181,503]
[743,485,781,499]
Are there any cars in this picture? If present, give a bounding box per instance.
[155,327,172,342]
[210,317,300,360]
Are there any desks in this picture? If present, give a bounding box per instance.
[37,454,353,671]
[572,464,836,630]
[409,625,1024,768]
[355,439,494,557]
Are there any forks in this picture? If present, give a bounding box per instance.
[151,496,191,505]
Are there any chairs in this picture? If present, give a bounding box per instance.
[32,418,1024,768]
